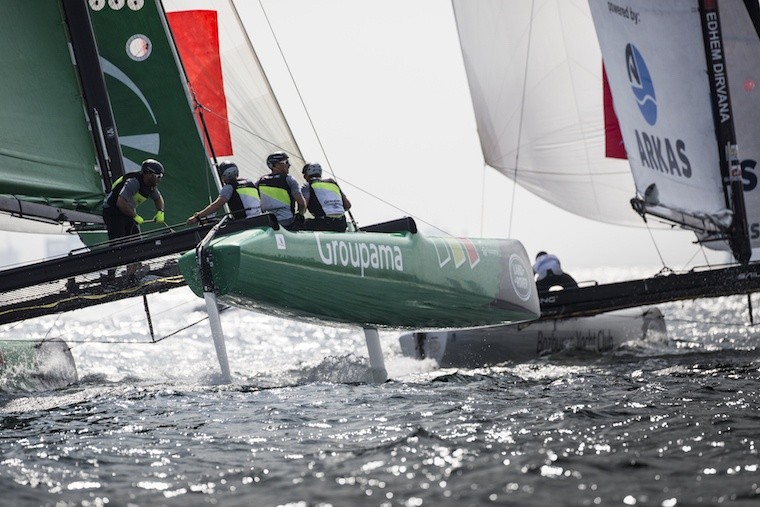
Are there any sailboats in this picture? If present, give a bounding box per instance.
[0,0,760,397]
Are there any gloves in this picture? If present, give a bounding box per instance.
[133,214,144,225]
[153,209,165,223]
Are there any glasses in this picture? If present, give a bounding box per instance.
[149,173,164,180]
[278,160,289,166]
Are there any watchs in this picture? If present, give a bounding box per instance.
[158,208,165,212]
[193,211,199,220]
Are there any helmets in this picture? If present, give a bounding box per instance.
[266,153,290,171]
[301,162,323,179]
[139,160,164,178]
[218,162,238,179]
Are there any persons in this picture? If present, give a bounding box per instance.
[102,158,165,278]
[186,152,351,233]
[532,251,563,281]
